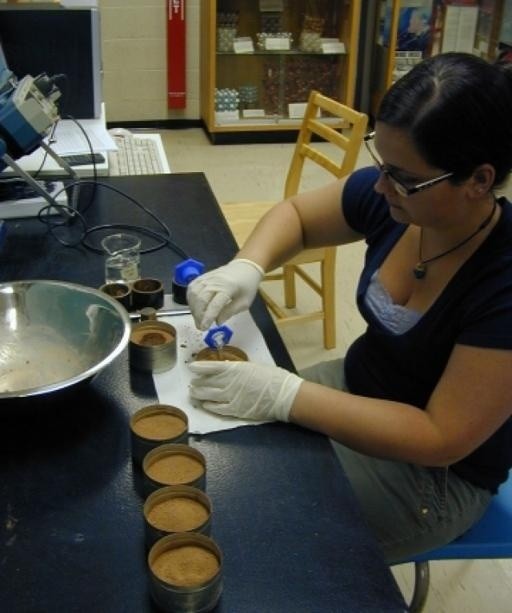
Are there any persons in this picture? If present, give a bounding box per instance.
[185,50,510,566]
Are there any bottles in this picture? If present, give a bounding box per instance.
[217,6,240,51]
[216,83,258,109]
[255,30,293,49]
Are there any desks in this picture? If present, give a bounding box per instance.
[0,169,409,612]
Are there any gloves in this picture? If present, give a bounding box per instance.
[188,360,303,423]
[187,259,265,330]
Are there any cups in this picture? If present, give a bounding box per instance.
[130,322,178,374]
[102,231,142,287]
[140,443,208,496]
[195,345,248,378]
[128,404,188,464]
[148,530,225,613]
[172,277,194,304]
[102,276,164,310]
[142,484,214,532]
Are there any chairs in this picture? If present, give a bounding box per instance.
[386,468,512,613]
[217,89,370,351]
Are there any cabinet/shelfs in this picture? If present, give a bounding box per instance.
[0,100,171,186]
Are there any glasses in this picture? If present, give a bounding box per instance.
[363,132,456,198]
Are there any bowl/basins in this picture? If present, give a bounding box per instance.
[0,277,133,400]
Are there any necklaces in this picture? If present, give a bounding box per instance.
[412,197,497,279]
